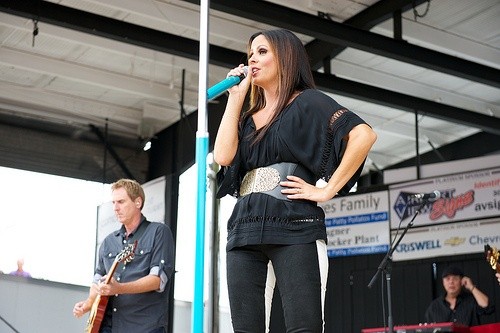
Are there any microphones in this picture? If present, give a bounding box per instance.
[412,190,441,201]
[207,66,252,100]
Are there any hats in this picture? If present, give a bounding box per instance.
[441,266,464,277]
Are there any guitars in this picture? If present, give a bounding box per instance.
[86,240,138,333]
[484,244,500,274]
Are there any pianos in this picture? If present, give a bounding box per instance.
[362,323,470,333]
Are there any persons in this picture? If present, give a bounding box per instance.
[73,179,174,333]
[424,268,492,325]
[213,29,377,333]
